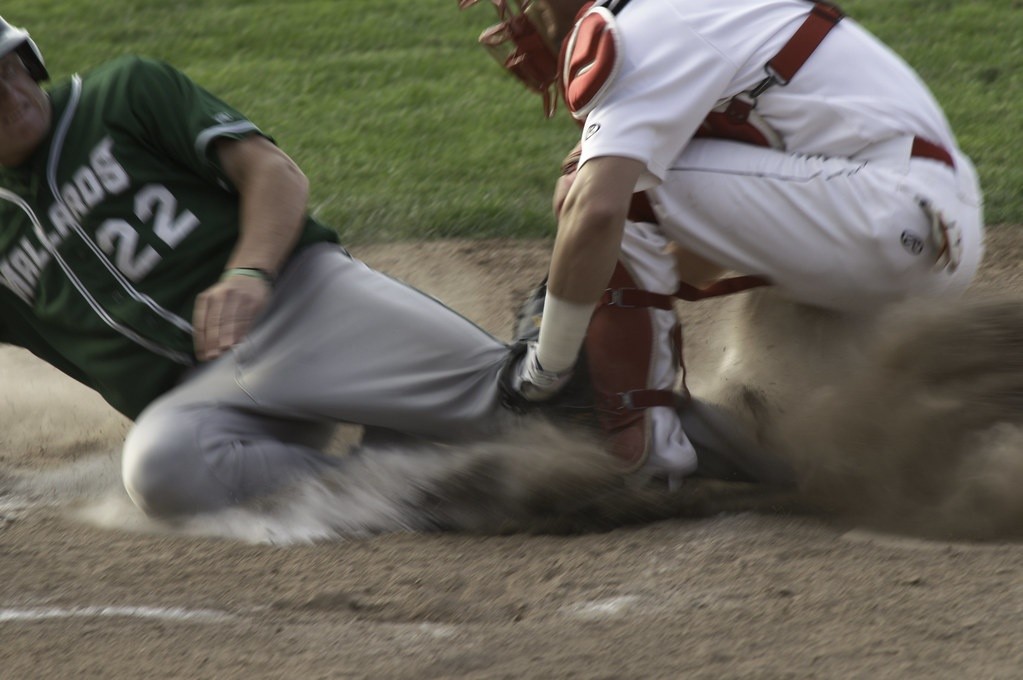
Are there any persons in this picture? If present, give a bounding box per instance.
[459,1,986,484]
[1,19,761,538]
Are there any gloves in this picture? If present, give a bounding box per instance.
[512,343,573,406]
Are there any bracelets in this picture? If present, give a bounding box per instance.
[219,267,276,292]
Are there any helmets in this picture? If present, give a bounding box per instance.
[0,14,51,87]
